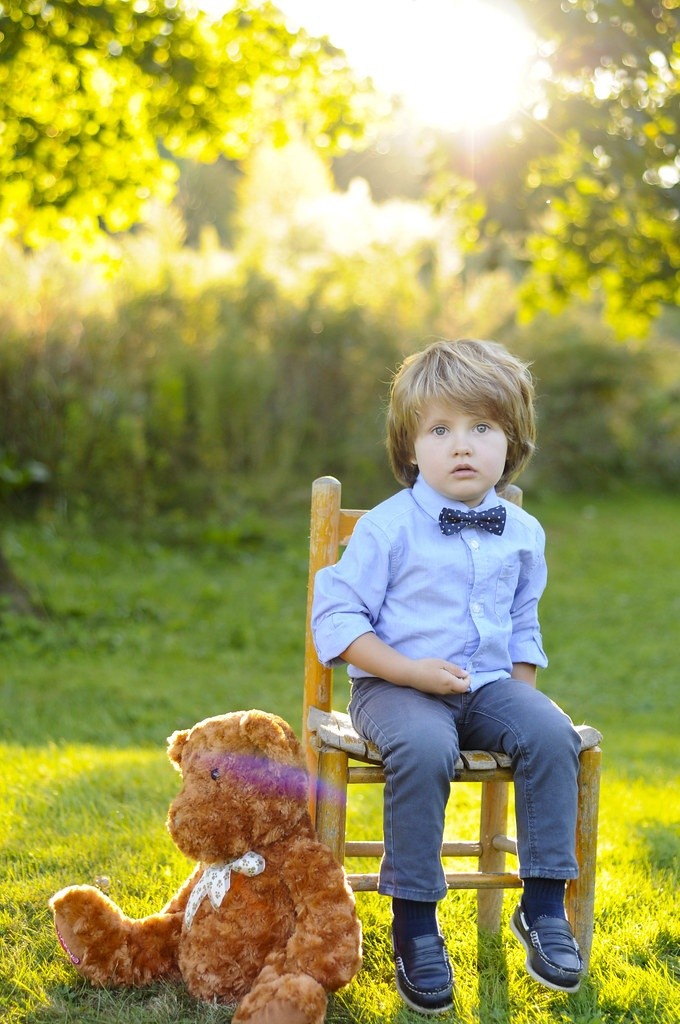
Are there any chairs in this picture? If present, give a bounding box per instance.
[302,477,602,975]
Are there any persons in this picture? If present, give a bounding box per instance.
[308,338,589,1014]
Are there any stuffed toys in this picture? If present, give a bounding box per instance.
[47,707,362,1024]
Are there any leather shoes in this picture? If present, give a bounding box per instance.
[392,921,456,1014]
[511,902,585,993]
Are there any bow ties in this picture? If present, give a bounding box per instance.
[438,505,506,536]
